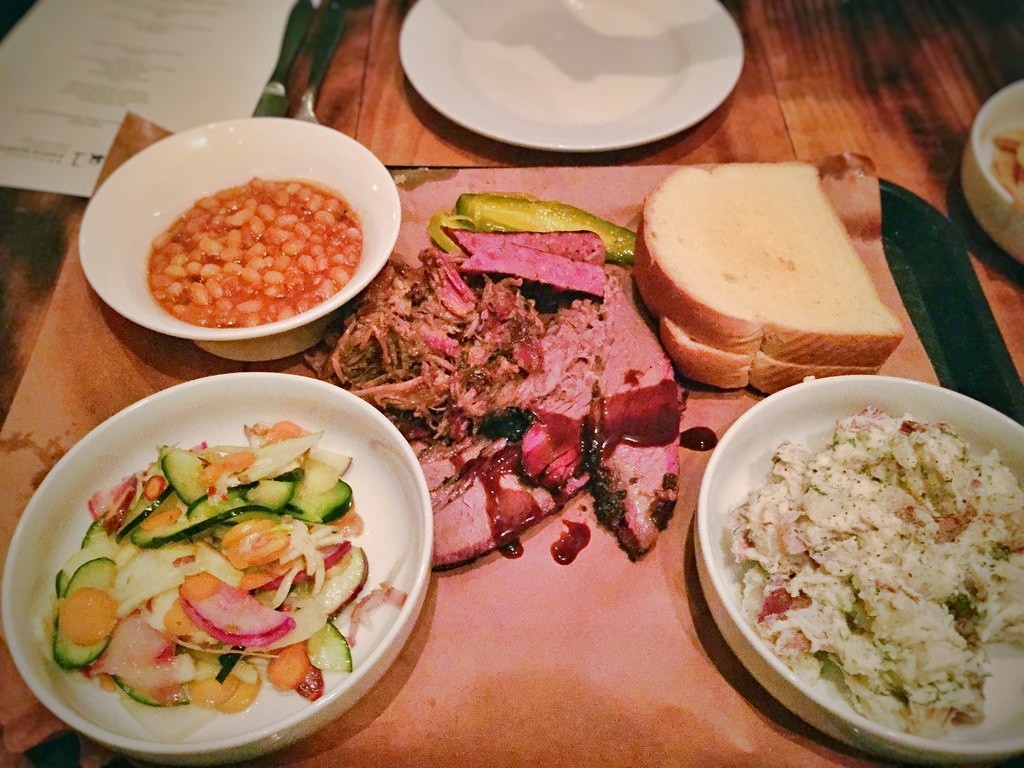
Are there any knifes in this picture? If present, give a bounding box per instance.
[245,0,315,119]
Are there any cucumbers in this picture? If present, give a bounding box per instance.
[455,193,637,265]
[49,447,354,704]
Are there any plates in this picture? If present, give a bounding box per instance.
[398,0,744,151]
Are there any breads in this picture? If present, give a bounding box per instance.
[633,160,903,393]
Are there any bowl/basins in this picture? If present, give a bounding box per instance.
[80,114,402,363]
[959,78,1024,269]
[1,371,436,764]
[690,375,1023,765]
